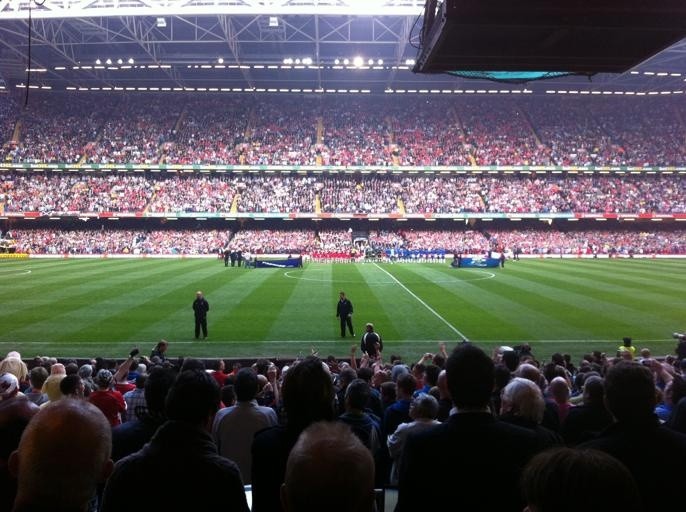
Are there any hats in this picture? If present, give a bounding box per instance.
[0,372,18,395]
[94,369,116,388]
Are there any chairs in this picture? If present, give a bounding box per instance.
[373,488,385,512]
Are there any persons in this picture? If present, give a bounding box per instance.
[336,291,356,338]
[0,338,686,511]
[192,290,210,339]
[0,81,686,268]
[360,323,385,358]
